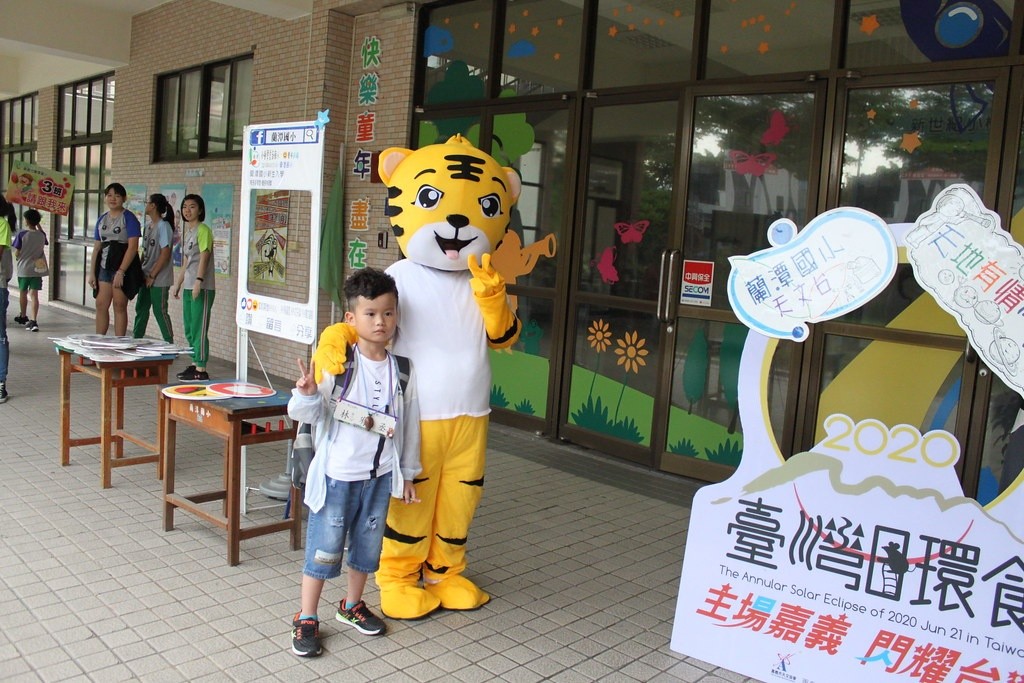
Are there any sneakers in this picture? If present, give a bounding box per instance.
[26,320,39,331]
[336,598,386,635]
[292,609,322,656]
[14,316,30,326]
[0,384,8,402]
[179,366,210,382]
[176,366,196,378]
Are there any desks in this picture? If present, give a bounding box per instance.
[158,380,301,568]
[54,340,181,490]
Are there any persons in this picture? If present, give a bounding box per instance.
[0,191,18,404]
[172,193,216,383]
[286,266,424,658]
[131,192,176,345]
[88,182,145,337]
[10,208,50,332]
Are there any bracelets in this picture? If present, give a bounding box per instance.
[196,277,204,282]
[116,267,127,278]
[149,274,156,280]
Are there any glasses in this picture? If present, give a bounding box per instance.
[143,200,153,206]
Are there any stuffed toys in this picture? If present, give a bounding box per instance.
[312,130,523,623]
